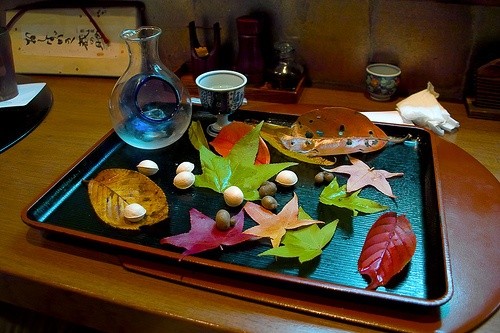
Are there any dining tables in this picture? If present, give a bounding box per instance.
[0,62,499,332]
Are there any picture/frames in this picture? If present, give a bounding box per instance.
[0,0,146,79]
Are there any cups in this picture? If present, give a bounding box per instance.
[366,64,400,102]
[0,26,19,101]
[196,70,248,137]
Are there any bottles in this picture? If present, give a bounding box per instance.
[269,40,303,89]
[109,26,192,148]
